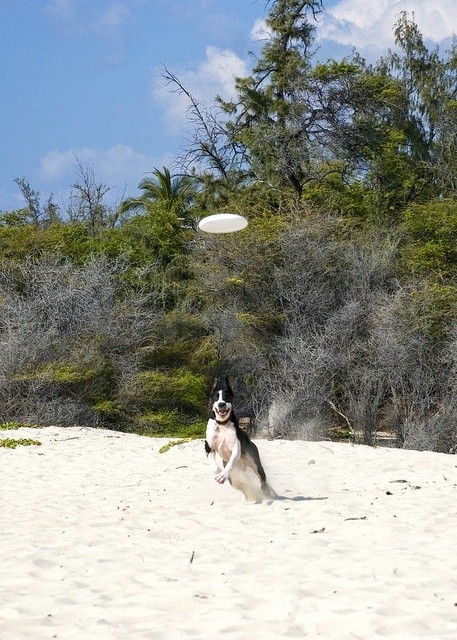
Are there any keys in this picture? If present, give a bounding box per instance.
[199,214,249,234]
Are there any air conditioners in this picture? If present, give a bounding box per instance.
[203,375,279,505]
